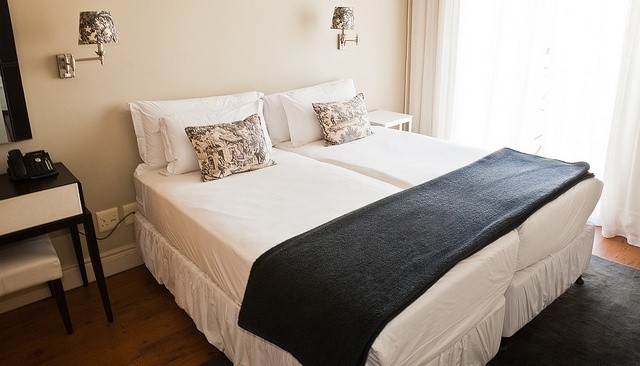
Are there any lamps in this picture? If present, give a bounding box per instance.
[331,7,358,47]
[56,10,118,80]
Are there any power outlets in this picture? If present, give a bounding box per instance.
[122,202,141,226]
[94,205,121,235]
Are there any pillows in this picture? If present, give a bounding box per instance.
[263,83,329,147]
[183,114,279,179]
[280,77,355,146]
[312,91,373,148]
[127,92,270,171]
[162,99,276,180]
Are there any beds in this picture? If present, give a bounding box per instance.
[128,75,605,366]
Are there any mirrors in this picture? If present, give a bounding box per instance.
[0,0,34,146]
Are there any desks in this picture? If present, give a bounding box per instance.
[367,108,413,137]
[0,161,113,323]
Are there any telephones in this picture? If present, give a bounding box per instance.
[6,149,59,181]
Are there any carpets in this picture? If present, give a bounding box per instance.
[467,253,639,366]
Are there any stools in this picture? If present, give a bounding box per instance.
[1,230,75,335]
